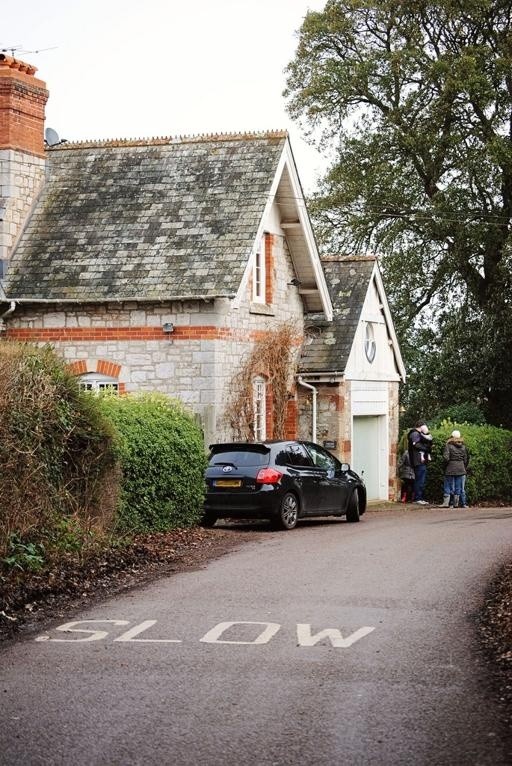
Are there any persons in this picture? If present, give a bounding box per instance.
[417,424,433,464]
[447,443,471,509]
[407,420,431,506]
[435,430,469,509]
[446,474,469,509]
[397,449,416,504]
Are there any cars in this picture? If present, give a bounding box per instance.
[203,435,366,528]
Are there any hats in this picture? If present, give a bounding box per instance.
[451,430,461,438]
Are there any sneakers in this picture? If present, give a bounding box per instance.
[422,500,430,505]
[415,499,426,505]
[462,503,470,508]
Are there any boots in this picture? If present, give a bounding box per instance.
[401,490,407,503]
[436,494,450,508]
[411,491,416,503]
[453,494,460,508]
